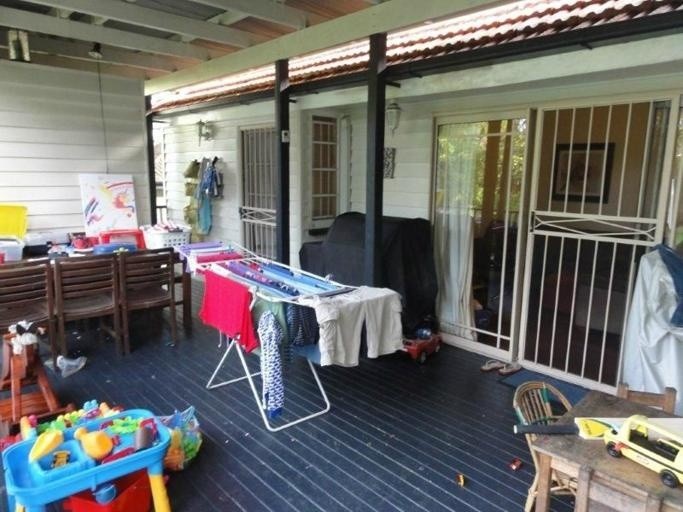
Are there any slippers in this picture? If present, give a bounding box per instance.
[481,360,506,372]
[499,363,523,376]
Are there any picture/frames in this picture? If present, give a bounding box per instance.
[551,141,616,204]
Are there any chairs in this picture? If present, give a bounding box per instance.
[124,245,178,356]
[615,384,680,412]
[53,252,121,355]
[512,381,579,511]
[0,259,59,373]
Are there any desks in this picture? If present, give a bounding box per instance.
[532,391,683,512]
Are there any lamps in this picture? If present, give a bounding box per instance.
[386,99,404,132]
[88,42,104,58]
